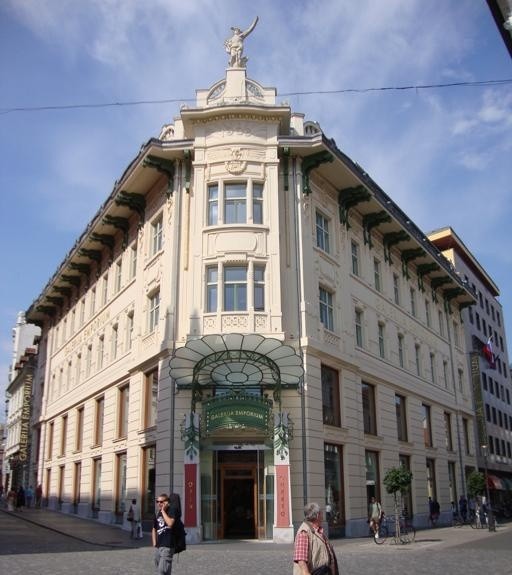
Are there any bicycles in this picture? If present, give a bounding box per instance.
[452,508,496,530]
[374,515,416,545]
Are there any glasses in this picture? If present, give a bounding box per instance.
[156,500,164,504]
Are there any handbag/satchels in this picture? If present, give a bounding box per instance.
[311,565,331,575]
[127,510,134,521]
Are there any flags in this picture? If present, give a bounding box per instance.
[482,337,497,369]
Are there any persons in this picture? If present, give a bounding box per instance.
[293,503,339,575]
[428,493,487,528]
[152,494,176,575]
[128,499,139,540]
[5,484,42,512]
[367,496,382,536]
[226,16,259,67]
